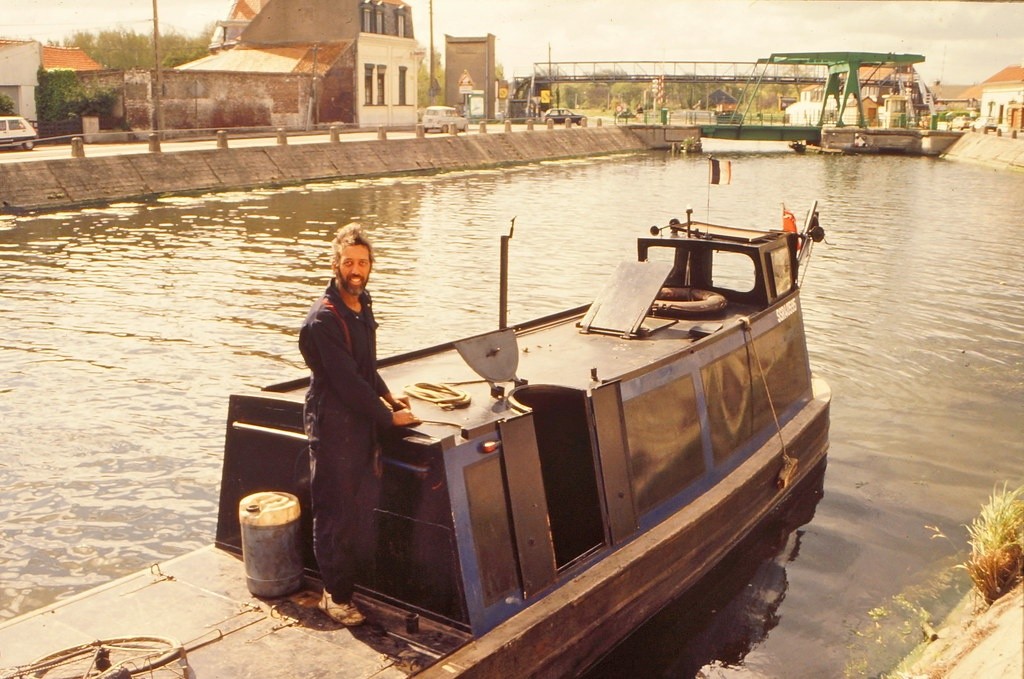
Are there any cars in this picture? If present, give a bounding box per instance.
[714,110,744,125]
[0,116,39,150]
[971,117,998,131]
[618,110,636,118]
[947,117,976,130]
[542,108,586,126]
[422,106,468,134]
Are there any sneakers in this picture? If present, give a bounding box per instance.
[317,588,367,626]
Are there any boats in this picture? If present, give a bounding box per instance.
[0,205,832,679]
[839,143,879,156]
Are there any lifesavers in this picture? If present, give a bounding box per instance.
[783,212,800,251]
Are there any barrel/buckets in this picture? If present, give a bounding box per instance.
[239,491,305,599]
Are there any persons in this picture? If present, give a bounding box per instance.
[297,223,422,627]
